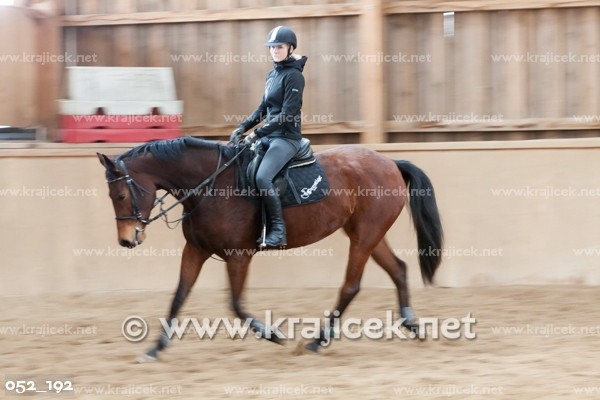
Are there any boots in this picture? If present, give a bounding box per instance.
[264,186,287,247]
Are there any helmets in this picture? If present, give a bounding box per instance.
[263,27,297,47]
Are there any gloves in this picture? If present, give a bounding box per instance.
[243,132,258,146]
[230,125,242,142]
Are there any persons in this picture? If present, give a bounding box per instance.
[230,26,309,247]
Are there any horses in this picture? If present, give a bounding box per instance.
[95,136,444,363]
